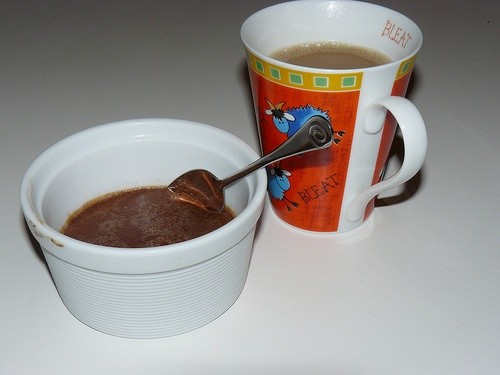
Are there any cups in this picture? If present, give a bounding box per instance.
[241,0,424,235]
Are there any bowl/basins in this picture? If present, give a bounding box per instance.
[20,118,268,338]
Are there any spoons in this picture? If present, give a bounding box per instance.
[166,115,334,213]
